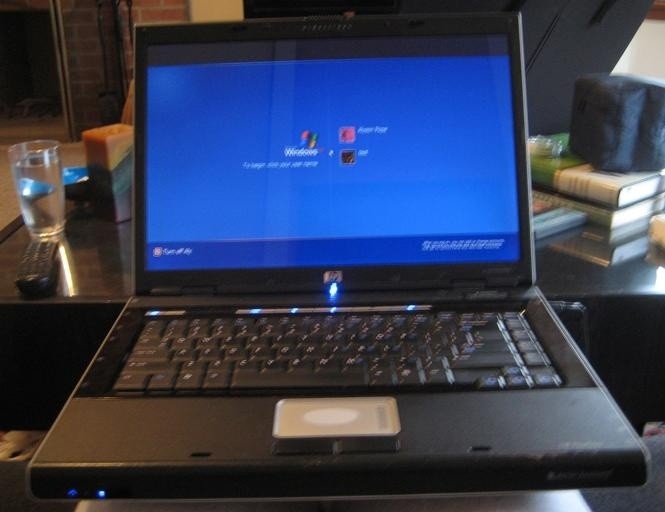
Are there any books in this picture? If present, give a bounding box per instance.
[552,225,650,269]
[531,131,665,208]
[531,188,665,230]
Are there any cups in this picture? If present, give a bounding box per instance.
[7,140,67,240]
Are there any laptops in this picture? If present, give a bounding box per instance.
[24,11,652,501]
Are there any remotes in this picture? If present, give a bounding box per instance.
[12,241,60,294]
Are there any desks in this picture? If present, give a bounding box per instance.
[2,168,665,301]
